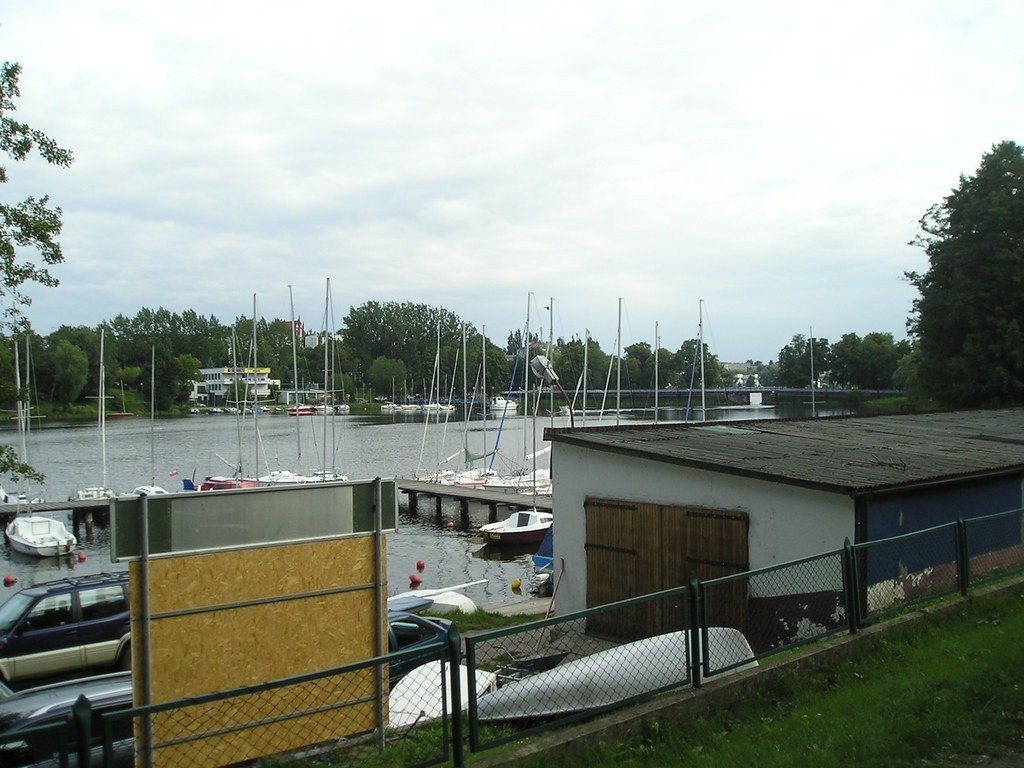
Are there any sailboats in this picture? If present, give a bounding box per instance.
[5,302,78,557]
[69,329,116,500]
[0,331,43,505]
[174,275,827,512]
[118,343,170,496]
[477,383,554,545]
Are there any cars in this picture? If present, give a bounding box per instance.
[386,610,463,689]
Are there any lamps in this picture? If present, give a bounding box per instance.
[530,355,575,432]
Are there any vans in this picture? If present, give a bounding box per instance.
[389,660,497,727]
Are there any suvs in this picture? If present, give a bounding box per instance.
[0,570,133,682]
[1,669,134,768]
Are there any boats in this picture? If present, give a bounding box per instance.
[388,587,478,616]
[524,522,553,597]
[476,626,761,722]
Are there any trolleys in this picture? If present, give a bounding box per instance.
[493,639,571,672]
